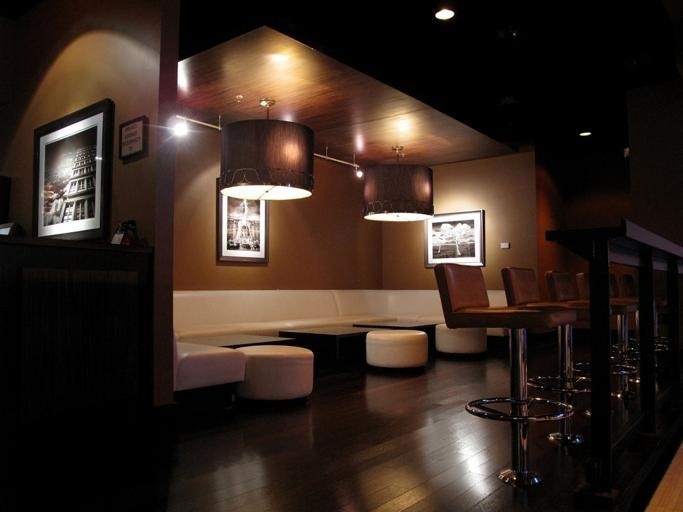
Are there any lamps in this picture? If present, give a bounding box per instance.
[220,98,314,200]
[361,145,433,221]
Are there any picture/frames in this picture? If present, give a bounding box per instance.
[119,115,147,159]
[32,97,115,241]
[217,177,269,264]
[424,209,485,268]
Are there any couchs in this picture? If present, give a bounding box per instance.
[174,290,509,408]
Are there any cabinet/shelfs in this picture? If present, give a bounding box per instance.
[3,237,155,512]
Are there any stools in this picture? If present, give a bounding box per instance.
[546,269,668,402]
[366,330,429,376]
[434,264,576,486]
[435,323,487,354]
[234,345,315,403]
[500,266,592,450]
